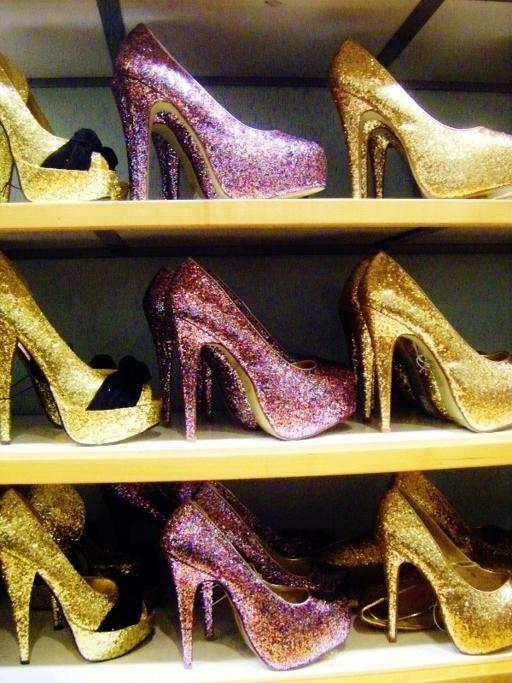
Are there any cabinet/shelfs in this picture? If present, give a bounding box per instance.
[0,0,512,683]
